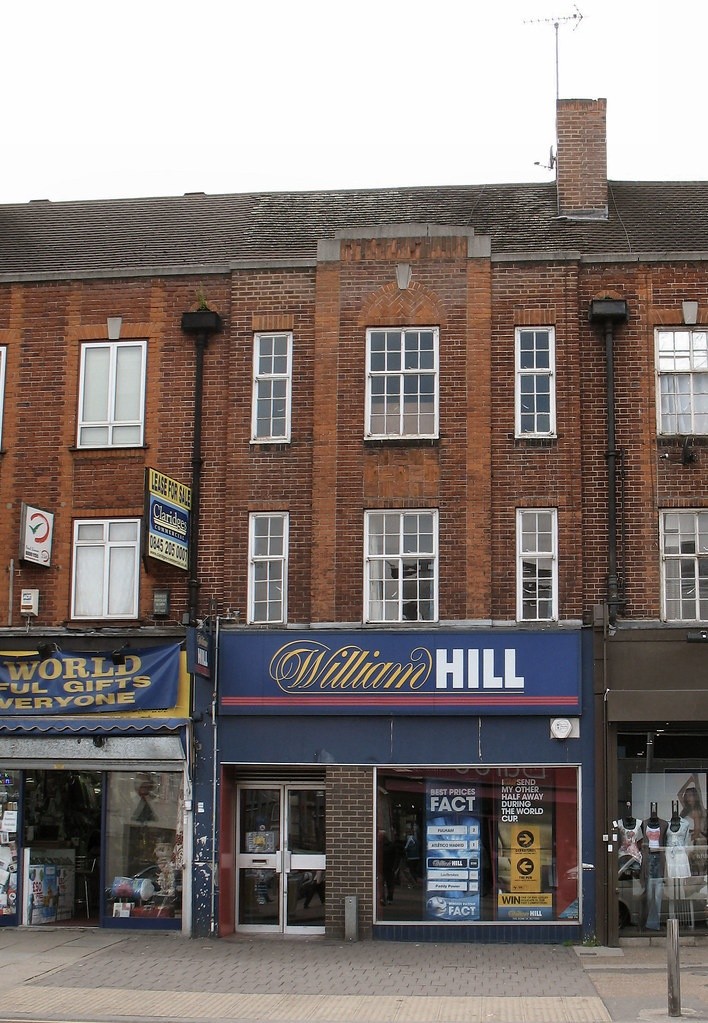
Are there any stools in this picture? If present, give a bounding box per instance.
[74,858,98,919]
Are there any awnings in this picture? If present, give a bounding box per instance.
[0,716,188,737]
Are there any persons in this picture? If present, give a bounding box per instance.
[677,776,708,885]
[616,814,692,931]
[378,830,397,906]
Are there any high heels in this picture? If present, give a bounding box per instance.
[266,899,274,903]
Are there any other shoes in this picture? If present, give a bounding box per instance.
[304,905,310,908]
[388,901,392,904]
[379,898,386,907]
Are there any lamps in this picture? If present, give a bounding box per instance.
[109,641,129,665]
[37,641,58,659]
